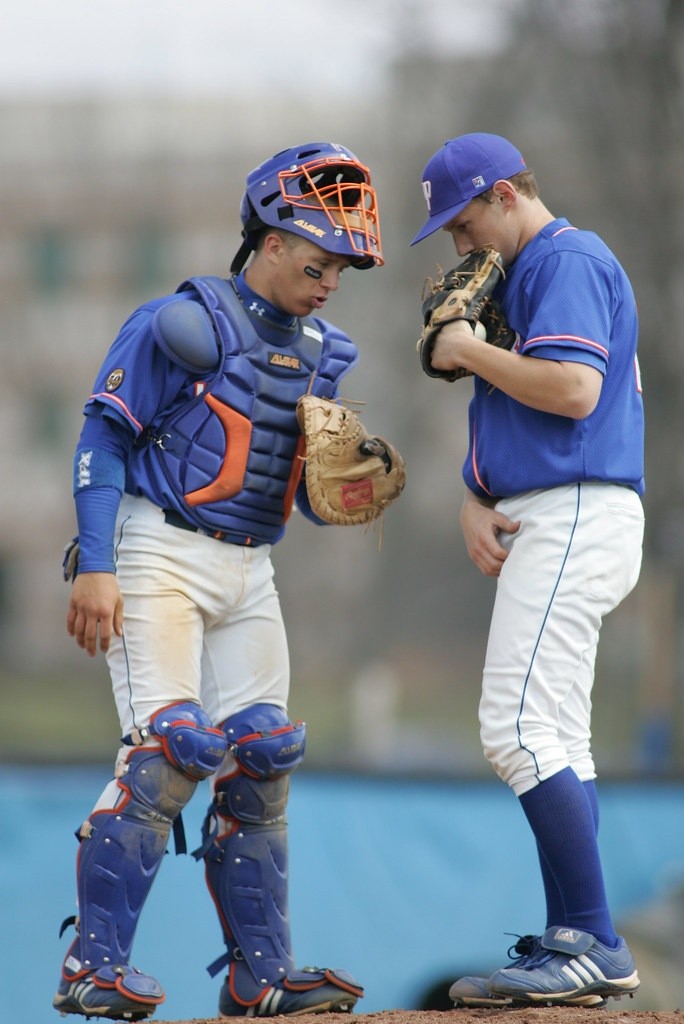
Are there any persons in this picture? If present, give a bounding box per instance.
[56,141,404,1023]
[412,132,647,1007]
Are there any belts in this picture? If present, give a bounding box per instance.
[164,509,225,540]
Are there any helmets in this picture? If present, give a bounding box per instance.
[239,141,386,271]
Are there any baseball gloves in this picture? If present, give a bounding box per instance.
[289,379,414,533]
[414,243,522,387]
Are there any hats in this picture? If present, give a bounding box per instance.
[410,132,526,246]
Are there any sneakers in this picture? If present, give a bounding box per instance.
[218,967,357,1017]
[492,927,641,1001]
[449,935,608,1006]
[52,939,158,1017]
